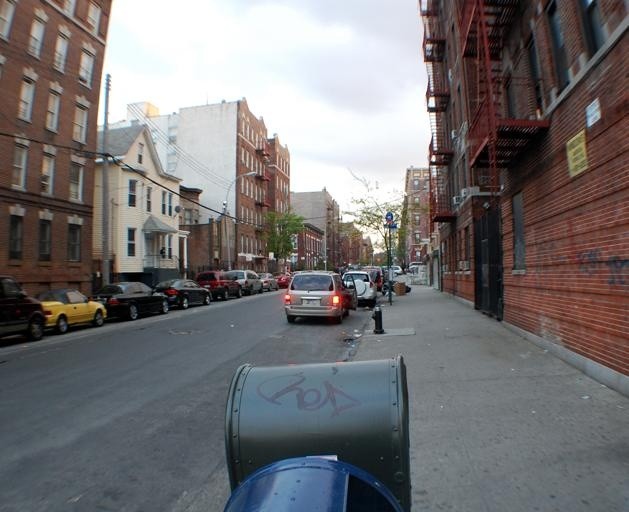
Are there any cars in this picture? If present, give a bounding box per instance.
[283,261,424,324]
[1,262,290,348]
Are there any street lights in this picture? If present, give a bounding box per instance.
[324,215,341,268]
[223,171,257,272]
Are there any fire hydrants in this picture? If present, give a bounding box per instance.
[371,304,385,335]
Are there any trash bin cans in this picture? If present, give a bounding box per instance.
[222,352,411,512]
